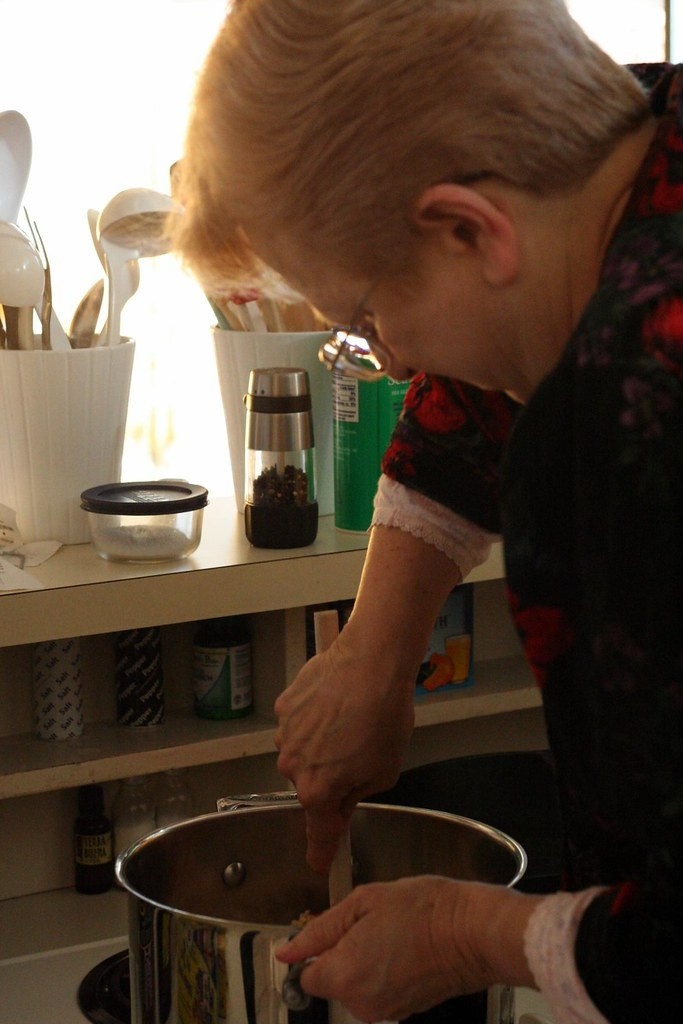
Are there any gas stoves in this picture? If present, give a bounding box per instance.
[1,935,558,1024]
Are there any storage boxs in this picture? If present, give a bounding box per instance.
[329,583,474,694]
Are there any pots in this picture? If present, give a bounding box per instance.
[114,797,528,1024]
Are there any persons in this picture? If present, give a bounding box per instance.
[177,0,682,1023]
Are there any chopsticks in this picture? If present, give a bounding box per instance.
[210,289,338,331]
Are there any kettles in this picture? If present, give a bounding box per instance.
[217,793,299,813]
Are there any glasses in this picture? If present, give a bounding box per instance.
[318,231,415,380]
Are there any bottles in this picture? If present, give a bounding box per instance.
[157,768,196,830]
[112,773,157,892]
[73,783,112,895]
[244,366,319,548]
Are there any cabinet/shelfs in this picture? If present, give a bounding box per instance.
[0,497,541,966]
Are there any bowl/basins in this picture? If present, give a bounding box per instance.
[80,482,208,563]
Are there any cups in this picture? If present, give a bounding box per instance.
[2,341,131,545]
[215,321,337,517]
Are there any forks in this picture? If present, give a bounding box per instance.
[17,205,52,350]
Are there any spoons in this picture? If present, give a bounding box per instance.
[0,220,72,349]
[87,208,141,348]
[69,278,105,349]
